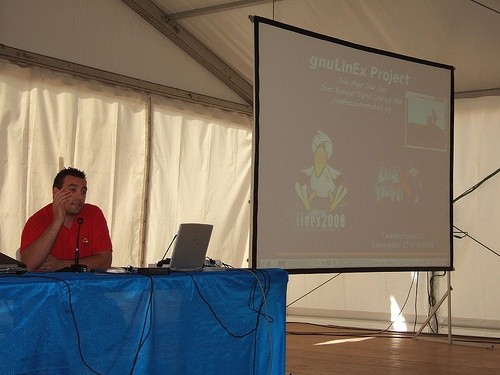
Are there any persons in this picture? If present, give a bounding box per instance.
[16,166,113,275]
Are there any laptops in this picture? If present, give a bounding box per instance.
[170,223,214,272]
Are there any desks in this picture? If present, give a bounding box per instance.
[0,269,288,375]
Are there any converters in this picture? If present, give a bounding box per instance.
[139,268,169,274]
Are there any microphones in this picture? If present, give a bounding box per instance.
[71,217,87,272]
